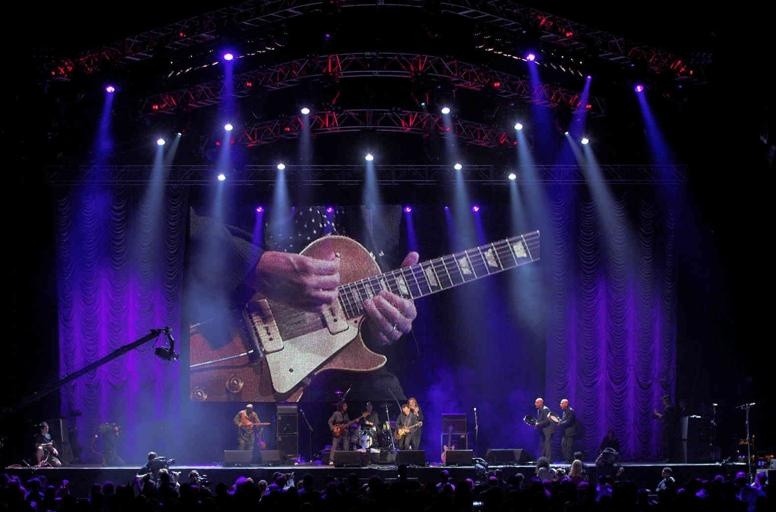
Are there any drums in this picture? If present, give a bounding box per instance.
[547,411,560,423]
[523,415,536,427]
[360,431,378,448]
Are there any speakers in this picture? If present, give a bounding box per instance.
[224,450,253,465]
[396,449,426,467]
[441,413,468,433]
[260,449,287,464]
[277,405,299,458]
[485,448,531,466]
[333,450,369,467]
[446,450,474,466]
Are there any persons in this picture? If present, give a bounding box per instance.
[328,402,356,466]
[554,399,578,464]
[395,403,419,451]
[187,209,416,400]
[33,420,61,467]
[600,430,620,454]
[361,401,380,449]
[531,397,557,463]
[655,395,679,463]
[233,403,261,448]
[406,397,425,450]
[673,397,695,452]
[1,451,776,511]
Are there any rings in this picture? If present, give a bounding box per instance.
[388,324,398,337]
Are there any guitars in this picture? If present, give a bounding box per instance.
[191,231,539,403]
[440,427,455,463]
[394,421,422,440]
[333,412,369,437]
